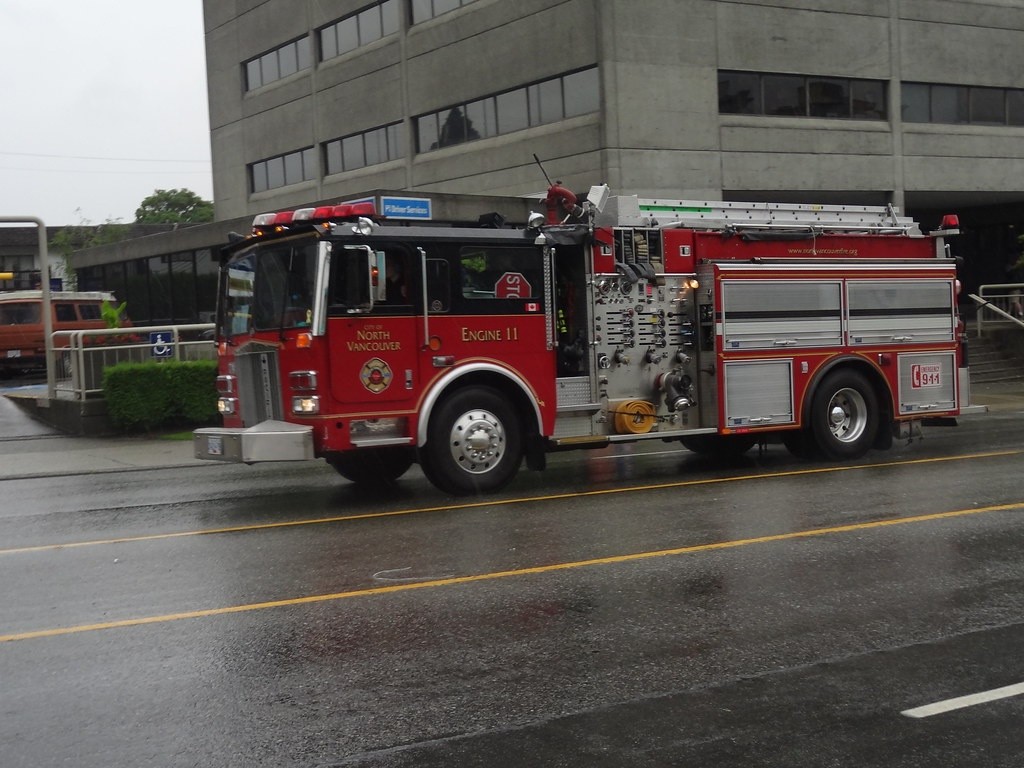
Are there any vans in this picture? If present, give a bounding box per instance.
[0,290,141,372]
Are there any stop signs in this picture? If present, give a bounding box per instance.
[494,272,531,299]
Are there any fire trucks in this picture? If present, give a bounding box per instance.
[190,150,988,498]
